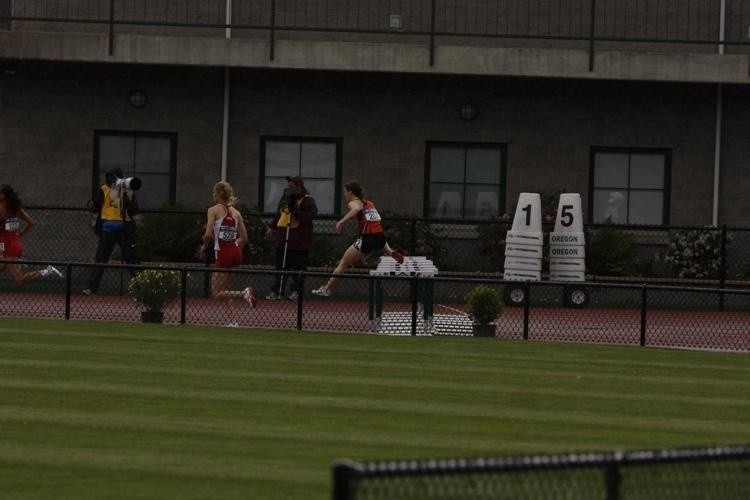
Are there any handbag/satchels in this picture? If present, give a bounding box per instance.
[121,192,138,238]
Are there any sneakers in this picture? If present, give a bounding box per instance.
[82,288,95,297]
[265,292,280,300]
[288,292,299,300]
[391,250,404,264]
[47,265,65,280]
[226,322,239,328]
[312,286,331,297]
[243,287,258,309]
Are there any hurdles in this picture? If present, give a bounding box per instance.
[369,255,439,335]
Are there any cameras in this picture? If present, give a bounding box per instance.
[115,176,141,192]
[282,188,297,204]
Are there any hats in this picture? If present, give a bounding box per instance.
[286,175,304,186]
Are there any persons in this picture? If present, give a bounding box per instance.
[81,167,144,298]
[312,183,405,297]
[0,183,64,289]
[199,182,258,328]
[266,176,317,302]
